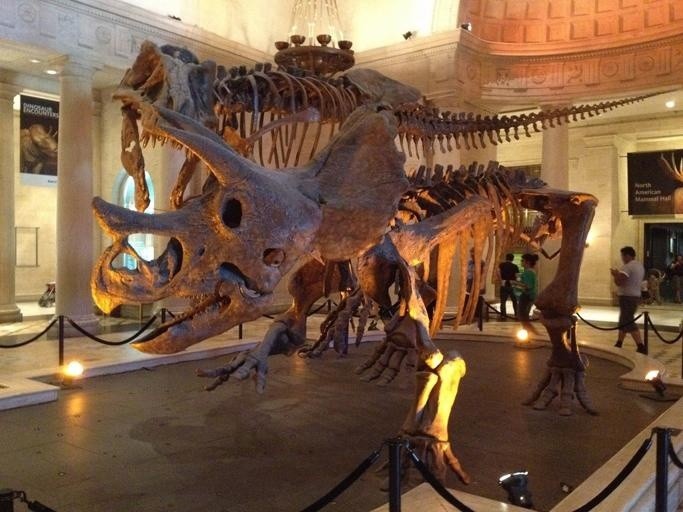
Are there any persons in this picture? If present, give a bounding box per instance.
[510,253,540,320]
[463,246,485,317]
[494,253,518,320]
[646,273,663,305]
[669,254,683,303]
[608,245,647,354]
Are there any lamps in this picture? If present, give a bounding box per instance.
[493,466,538,511]
[459,22,472,32]
[402,31,412,41]
[271,0,356,81]
[644,369,667,395]
